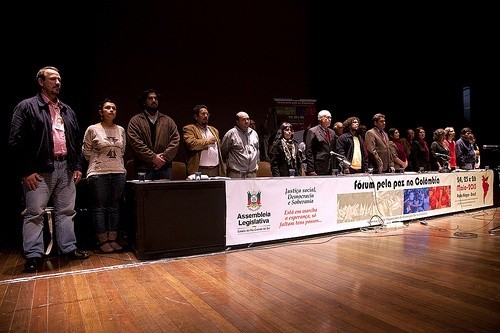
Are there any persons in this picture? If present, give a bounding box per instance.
[333,122,343,136]
[337,117,368,174]
[269,122,306,177]
[8,66,90,273]
[456,128,482,170]
[359,125,367,137]
[405,129,415,149]
[306,110,339,175]
[431,129,450,171]
[444,127,457,168]
[365,114,395,174]
[410,127,434,173]
[182,105,224,177]
[221,112,260,178]
[390,128,408,172]
[127,89,180,180]
[81,100,127,252]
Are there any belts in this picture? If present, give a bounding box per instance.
[199,164,219,169]
[54,156,66,161]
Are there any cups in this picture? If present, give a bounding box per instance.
[332,169,338,177]
[138,173,146,182]
[196,172,201,180]
[290,169,295,178]
[420,168,424,173]
[485,166,489,171]
[368,168,373,175]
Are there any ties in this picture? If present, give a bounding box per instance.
[380,130,386,143]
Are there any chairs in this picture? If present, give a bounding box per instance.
[170,161,188,179]
[255,161,273,177]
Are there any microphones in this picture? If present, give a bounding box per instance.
[483,145,500,149]
[434,152,449,157]
[330,151,346,159]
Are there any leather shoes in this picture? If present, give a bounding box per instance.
[61,248,89,259]
[25,257,42,273]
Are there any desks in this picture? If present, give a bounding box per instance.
[127,167,493,254]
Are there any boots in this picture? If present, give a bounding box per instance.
[97,230,122,253]
